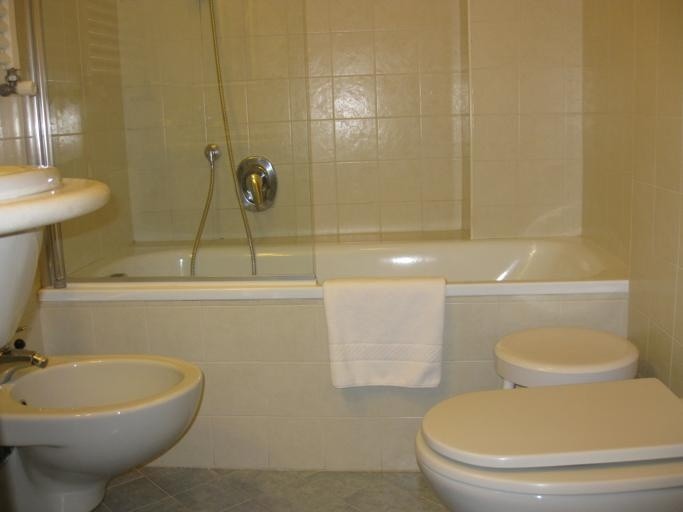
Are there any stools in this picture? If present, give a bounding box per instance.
[491,329,641,394]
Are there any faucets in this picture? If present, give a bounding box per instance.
[0,350,49,370]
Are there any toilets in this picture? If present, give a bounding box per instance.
[415,378,683,512]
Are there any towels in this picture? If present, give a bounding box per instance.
[323,275,450,388]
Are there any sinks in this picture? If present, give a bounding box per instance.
[0,164,204,348]
[0,354,204,483]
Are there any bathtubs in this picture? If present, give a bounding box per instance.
[74,239,629,473]
